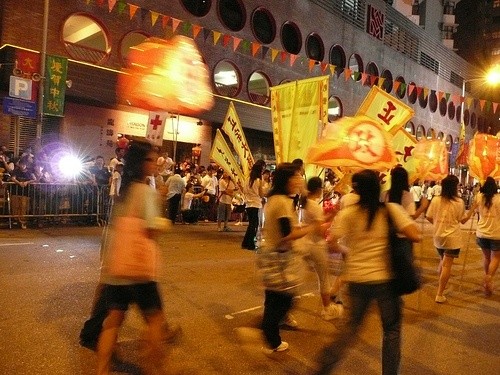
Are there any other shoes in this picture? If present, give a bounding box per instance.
[240,237,264,250]
[321,302,344,319]
[330,294,343,304]
[279,316,297,328]
[435,295,447,303]
[260,341,290,352]
[79,335,98,347]
[13,215,249,232]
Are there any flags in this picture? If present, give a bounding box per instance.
[269,76,329,167]
[468,133,500,184]
[353,85,415,134]
[401,138,448,179]
[455,122,469,170]
[388,128,418,164]
[208,128,247,192]
[308,115,396,169]
[222,101,254,178]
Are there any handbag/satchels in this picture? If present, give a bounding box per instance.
[384,203,423,295]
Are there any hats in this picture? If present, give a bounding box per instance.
[116,162,123,167]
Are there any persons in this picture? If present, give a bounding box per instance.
[231,188,246,226]
[217,169,235,231]
[255,168,273,240]
[307,169,423,375]
[425,175,478,302]
[340,189,361,210]
[430,182,442,196]
[254,162,321,355]
[299,176,344,320]
[475,176,500,297]
[292,158,309,200]
[269,170,275,189]
[410,178,422,210]
[94,140,180,375]
[241,159,267,250]
[421,180,429,197]
[427,180,436,200]
[116,132,130,149]
[0,144,225,229]
[458,182,500,210]
[381,167,429,264]
